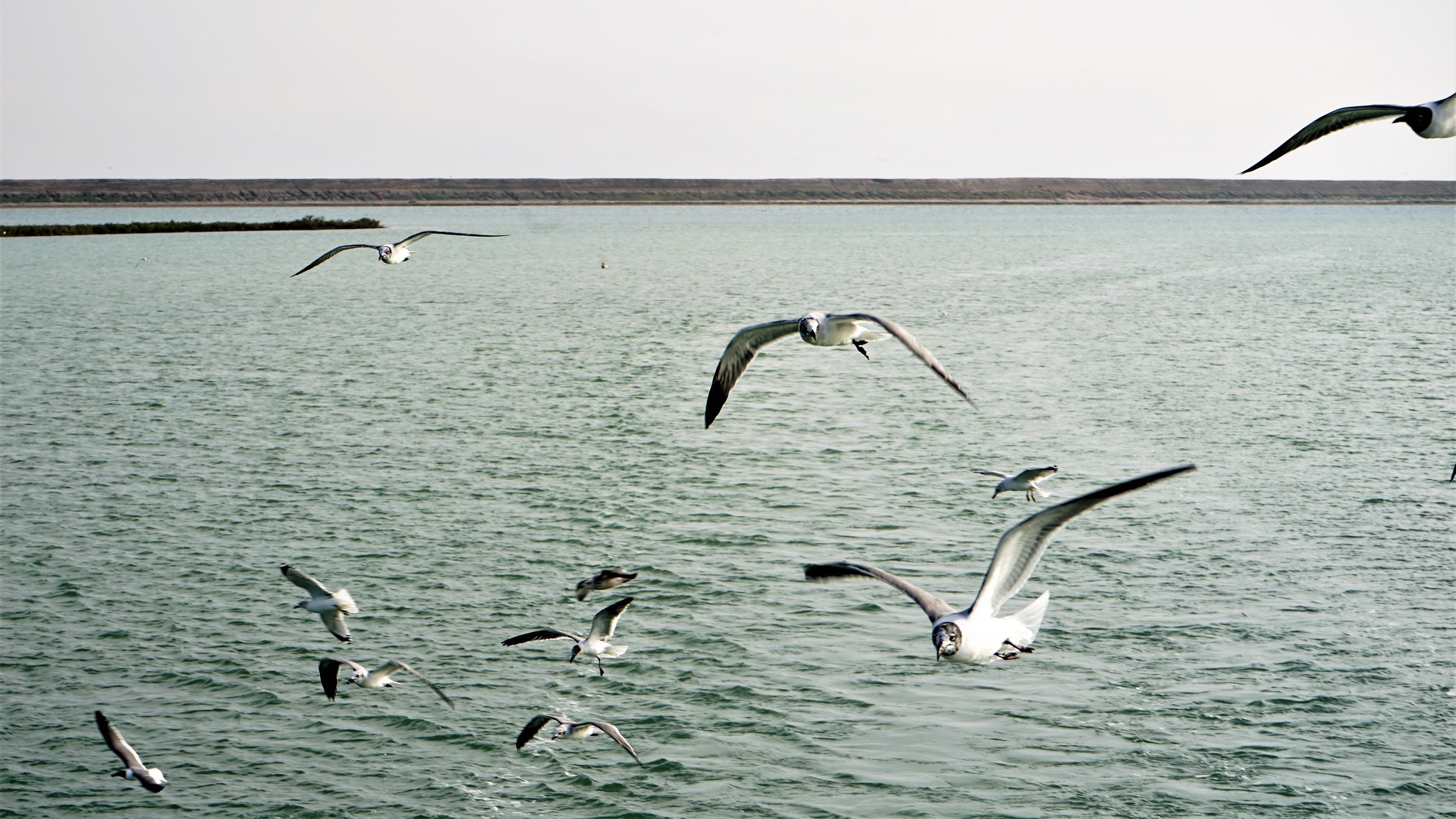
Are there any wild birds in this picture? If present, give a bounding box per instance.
[576,565,637,601]
[501,596,632,675]
[970,464,1059,503]
[279,562,359,645]
[1236,88,1456,176]
[801,463,1199,664]
[602,262,608,269]
[290,230,511,278]
[319,656,455,711]
[704,312,981,431]
[516,714,645,769]
[94,710,168,793]
